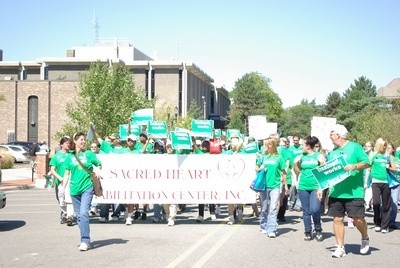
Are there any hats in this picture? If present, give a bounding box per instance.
[109,131,163,146]
[332,123,348,139]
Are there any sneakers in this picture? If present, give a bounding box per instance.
[332,245,346,258]
[304,232,311,240]
[316,231,323,241]
[360,235,370,254]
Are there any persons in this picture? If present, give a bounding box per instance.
[47,132,400,241]
[325,124,373,259]
[59,132,103,251]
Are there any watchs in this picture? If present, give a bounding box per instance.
[354,164,358,169]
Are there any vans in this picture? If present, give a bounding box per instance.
[6,141,40,156]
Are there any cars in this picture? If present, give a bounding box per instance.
[0,144,32,164]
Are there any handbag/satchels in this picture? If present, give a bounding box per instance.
[386,156,400,189]
[250,155,266,192]
[92,172,103,197]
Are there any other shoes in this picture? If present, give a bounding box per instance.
[262,229,278,234]
[79,243,88,251]
[67,219,72,225]
[196,216,204,221]
[211,214,216,220]
[375,227,380,231]
[348,221,354,228]
[90,208,174,226]
[61,218,66,223]
[382,229,387,232]
[238,213,243,221]
[268,233,275,237]
[279,216,285,222]
[215,207,220,214]
[227,217,234,224]
